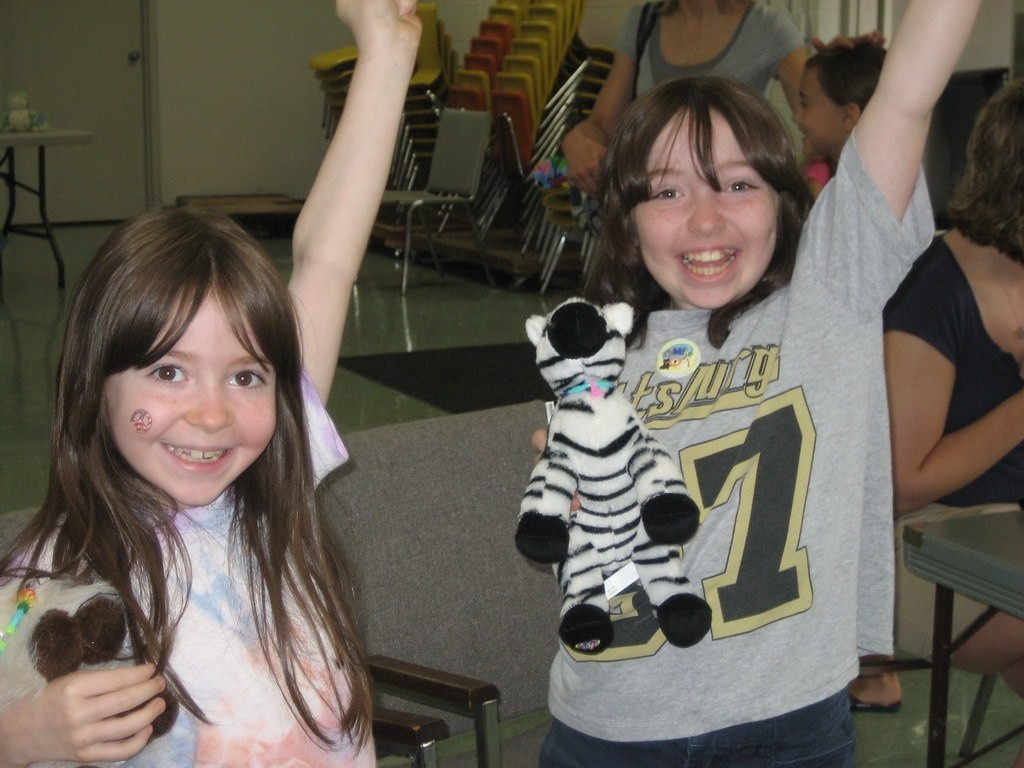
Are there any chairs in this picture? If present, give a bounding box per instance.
[310,0,618,296]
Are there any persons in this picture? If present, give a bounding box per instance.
[790,33,935,713]
[530,0,984,768]
[558,0,887,310]
[883,79,1024,698]
[0,0,422,768]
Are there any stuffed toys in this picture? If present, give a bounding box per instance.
[0,576,180,768]
[515,297,713,655]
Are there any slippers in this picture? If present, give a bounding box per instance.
[843,692,902,713]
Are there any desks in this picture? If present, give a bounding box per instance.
[905,499,1024,768]
[1,128,92,291]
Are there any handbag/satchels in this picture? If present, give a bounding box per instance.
[585,156,616,242]
